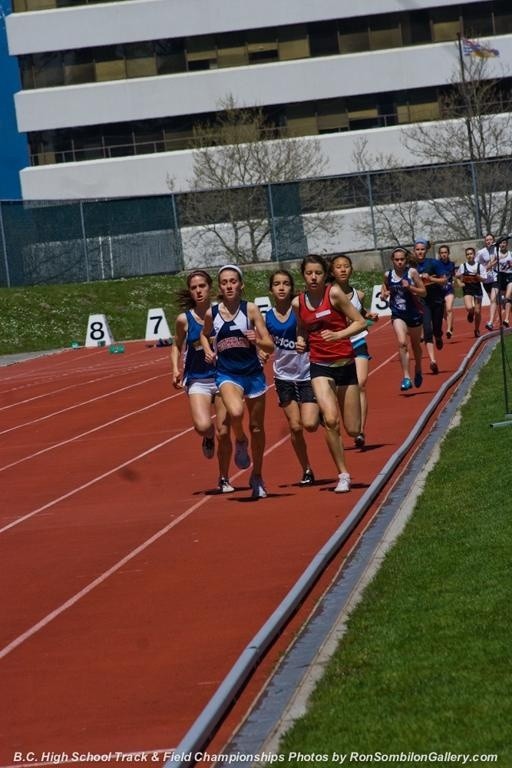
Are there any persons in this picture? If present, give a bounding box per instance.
[438,244,456,338]
[380,246,427,391]
[257,269,326,487]
[412,239,449,374]
[486,236,512,328]
[456,247,487,337]
[291,254,368,494]
[200,265,276,499]
[170,270,235,494]
[328,255,379,449]
[474,233,500,331]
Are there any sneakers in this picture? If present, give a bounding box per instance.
[202,436,215,458]
[249,473,267,498]
[217,476,234,492]
[447,331,452,339]
[503,320,510,328]
[334,473,352,493]
[234,438,251,469]
[415,366,423,388]
[355,434,365,449]
[474,330,480,336]
[430,360,438,374]
[435,337,443,350]
[400,378,413,390]
[486,321,493,330]
[468,313,474,323]
[299,468,314,485]
